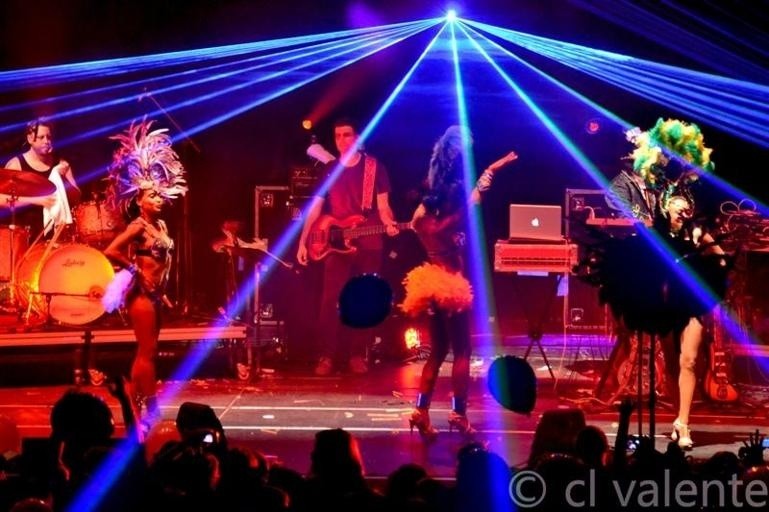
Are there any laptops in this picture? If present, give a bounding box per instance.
[508,204,561,244]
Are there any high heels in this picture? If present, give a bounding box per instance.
[409,410,439,435]
[448,411,477,435]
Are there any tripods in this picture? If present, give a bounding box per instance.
[0,196,48,323]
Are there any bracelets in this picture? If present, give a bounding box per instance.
[474,166,495,192]
[127,261,139,275]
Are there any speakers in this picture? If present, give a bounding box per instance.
[240,309,302,366]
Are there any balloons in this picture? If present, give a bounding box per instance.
[488,354,538,416]
[337,272,396,329]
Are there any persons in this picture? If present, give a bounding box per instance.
[0,388,769,510]
[104,186,176,425]
[296,118,401,378]
[409,124,519,442]
[666,193,695,242]
[591,169,660,410]
[0,119,83,245]
[671,226,727,453]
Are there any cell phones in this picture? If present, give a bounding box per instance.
[200,430,220,450]
[762,437,769,448]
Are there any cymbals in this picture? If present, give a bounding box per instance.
[1,167,55,196]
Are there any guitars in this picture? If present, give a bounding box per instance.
[306,215,413,261]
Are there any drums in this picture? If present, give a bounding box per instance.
[0,224,31,282]
[72,200,127,242]
[15,238,115,326]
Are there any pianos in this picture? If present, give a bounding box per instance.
[565,188,634,226]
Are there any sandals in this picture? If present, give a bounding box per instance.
[671,420,693,450]
